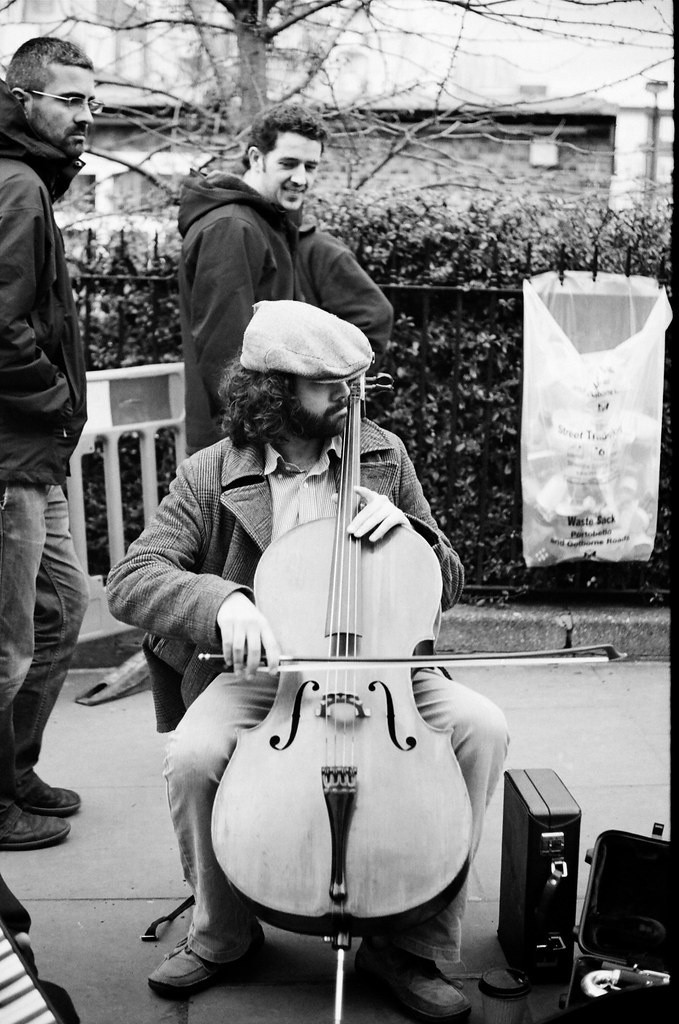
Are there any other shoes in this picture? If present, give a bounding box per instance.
[11,769,81,818]
[0,803,71,850]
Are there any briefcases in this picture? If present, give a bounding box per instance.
[558,823,671,1009]
[497,768,583,988]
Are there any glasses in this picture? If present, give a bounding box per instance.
[24,90,104,115]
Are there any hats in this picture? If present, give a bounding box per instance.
[240,300,372,385]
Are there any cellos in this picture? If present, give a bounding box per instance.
[211,370,476,1022]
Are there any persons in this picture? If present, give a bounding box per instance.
[296,213,393,419]
[177,104,329,458]
[105,301,510,1024]
[0,35,104,851]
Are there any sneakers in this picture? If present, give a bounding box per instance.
[355,936,471,1024]
[147,913,267,1001]
